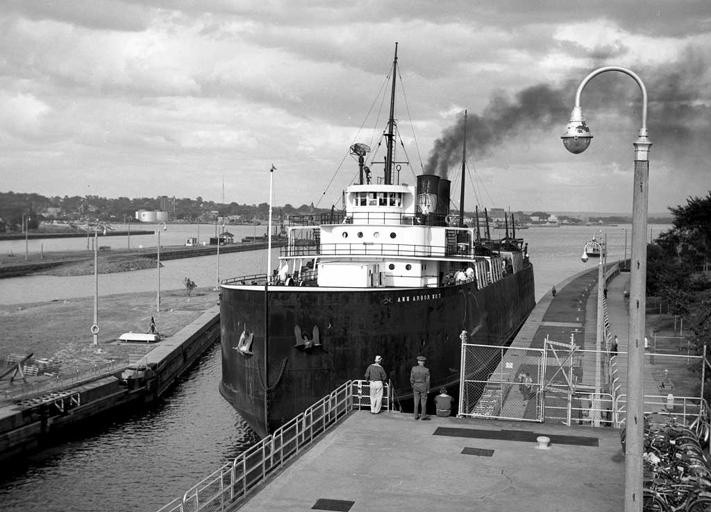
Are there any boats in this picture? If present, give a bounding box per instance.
[217,42,537,459]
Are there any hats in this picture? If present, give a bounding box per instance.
[374,355,383,362]
[416,356,427,361]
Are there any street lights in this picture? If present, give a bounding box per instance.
[91,224,107,345]
[156,221,167,312]
[25,215,32,261]
[560,66,653,511]
[581,230,607,427]
[267,162,277,277]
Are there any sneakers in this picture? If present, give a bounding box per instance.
[414,415,431,421]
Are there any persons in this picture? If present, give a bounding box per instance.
[364,355,388,414]
[518,369,534,401]
[441,264,475,288]
[434,386,454,417]
[613,334,619,355]
[410,356,429,420]
[271,269,294,286]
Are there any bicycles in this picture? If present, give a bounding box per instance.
[620,414,711,512]
